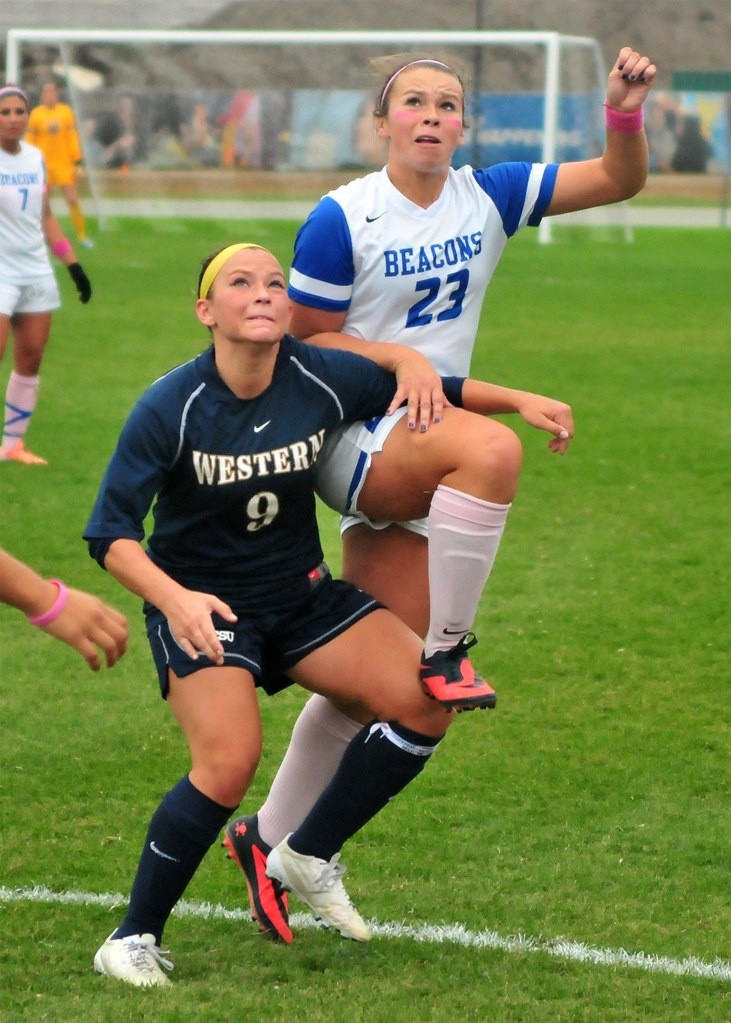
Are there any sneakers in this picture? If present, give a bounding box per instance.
[0,439,49,466]
[91,928,175,986]
[265,831,371,943]
[419,631,498,712]
[221,813,295,944]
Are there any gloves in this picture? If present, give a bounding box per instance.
[67,262,91,304]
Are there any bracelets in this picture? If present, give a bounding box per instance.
[27,579,67,626]
[605,98,644,134]
[51,237,72,258]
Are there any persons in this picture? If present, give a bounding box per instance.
[25,81,92,249]
[0,547,130,672]
[81,243,577,990]
[222,43,658,947]
[0,84,92,465]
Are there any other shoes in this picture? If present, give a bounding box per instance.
[80,235,94,249]
